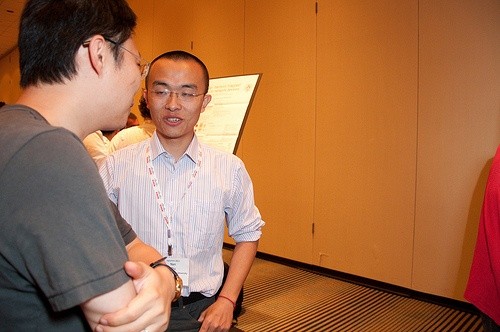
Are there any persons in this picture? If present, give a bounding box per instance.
[82,129,120,165]
[109,93,156,155]
[462,141,500,327]
[98,50,265,332]
[0,0,184,332]
[118,112,139,132]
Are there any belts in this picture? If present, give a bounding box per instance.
[171,291,204,307]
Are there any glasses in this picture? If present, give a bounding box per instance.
[146,88,205,100]
[81,38,150,80]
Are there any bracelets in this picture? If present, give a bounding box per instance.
[218,295,236,308]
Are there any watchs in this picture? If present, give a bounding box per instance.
[150,256,182,303]
